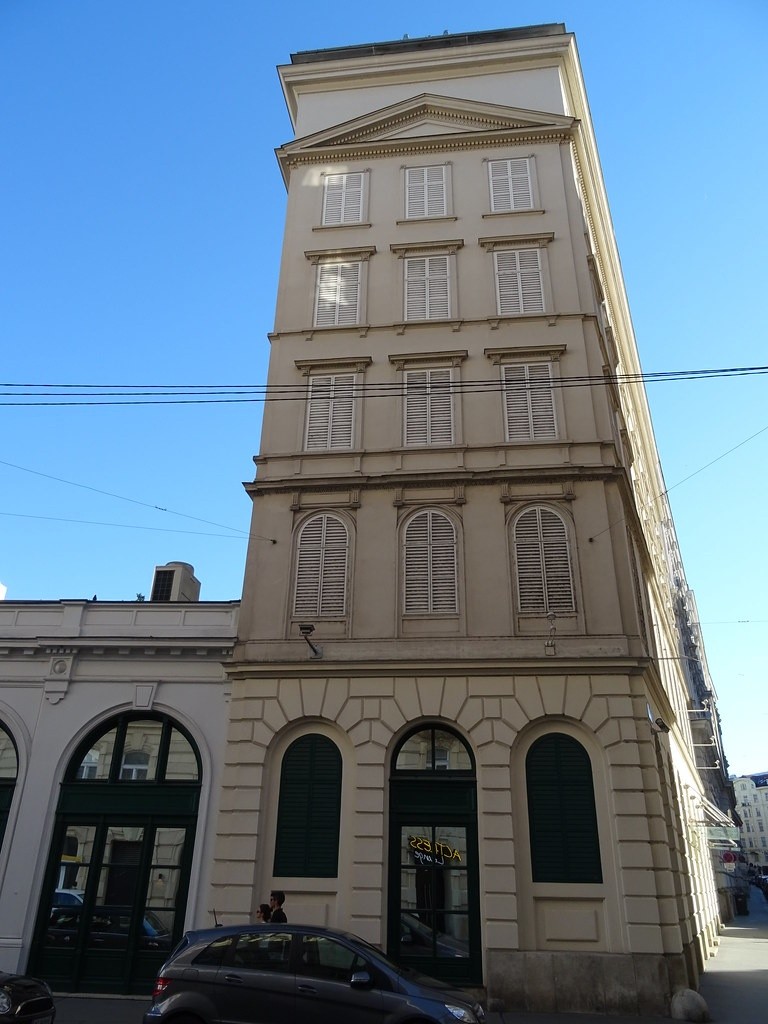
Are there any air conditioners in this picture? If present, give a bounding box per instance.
[150,565,201,601]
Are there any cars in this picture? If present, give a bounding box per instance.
[0,970,55,1024]
[50,889,86,918]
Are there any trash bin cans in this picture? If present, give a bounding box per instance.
[735,892,749,915]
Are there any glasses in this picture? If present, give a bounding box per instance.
[256,910,261,913]
[270,899,275,902]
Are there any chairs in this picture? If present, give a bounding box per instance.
[303,942,315,962]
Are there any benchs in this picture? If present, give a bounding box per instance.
[233,946,273,971]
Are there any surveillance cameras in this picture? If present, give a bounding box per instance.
[655,718,671,733]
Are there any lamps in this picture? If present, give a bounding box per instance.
[299,624,323,658]
[546,612,556,646]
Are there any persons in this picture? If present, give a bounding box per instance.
[256,890,288,923]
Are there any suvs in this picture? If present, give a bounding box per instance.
[400,912,470,958]
[143,924,490,1024]
[39,902,174,997]
